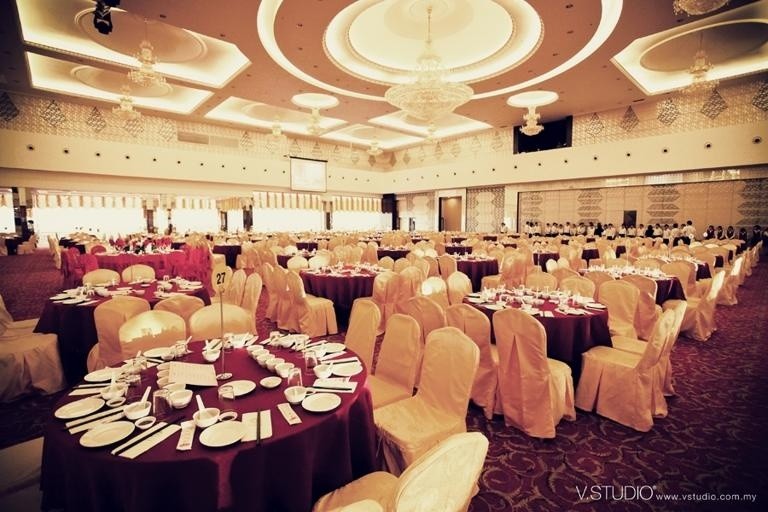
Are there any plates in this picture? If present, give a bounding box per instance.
[135,416,156,429]
[54,396,105,419]
[83,369,115,382]
[77,419,137,450]
[260,376,282,387]
[324,343,346,353]
[291,333,309,343]
[233,333,253,341]
[64,299,83,304]
[49,295,69,300]
[142,346,173,358]
[301,393,341,413]
[198,420,250,447]
[218,379,256,398]
[219,411,238,421]
[106,396,126,407]
[331,362,363,377]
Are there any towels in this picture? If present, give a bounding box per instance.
[311,378,358,394]
[320,356,362,367]
[65,404,128,435]
[584,305,605,312]
[154,290,186,301]
[201,338,223,351]
[67,382,111,397]
[122,356,165,369]
[296,339,329,353]
[240,408,273,443]
[540,311,554,318]
[178,280,204,290]
[259,333,291,346]
[110,421,182,460]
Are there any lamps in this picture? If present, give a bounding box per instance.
[110,95,134,116]
[386,8,474,133]
[366,139,378,155]
[311,109,321,133]
[680,50,719,90]
[126,41,162,88]
[273,125,284,137]
[518,104,542,135]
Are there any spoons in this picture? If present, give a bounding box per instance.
[195,394,212,423]
[205,339,212,355]
[133,384,153,421]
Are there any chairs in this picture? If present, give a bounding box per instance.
[274,264,298,333]
[83,269,120,286]
[444,304,503,420]
[527,273,557,292]
[634,259,659,271]
[648,257,665,268]
[491,308,577,439]
[153,295,205,336]
[189,304,252,336]
[0,319,35,337]
[620,274,664,342]
[446,271,472,304]
[673,259,701,296]
[584,271,614,302]
[559,275,595,299]
[314,432,488,512]
[310,256,332,267]
[379,255,392,268]
[414,259,428,274]
[287,271,339,335]
[79,254,98,274]
[46,230,741,262]
[0,435,47,488]
[438,254,459,275]
[420,276,450,309]
[373,327,480,497]
[0,332,65,402]
[224,269,246,306]
[0,295,39,327]
[363,315,421,409]
[612,300,688,397]
[348,271,394,336]
[557,258,569,267]
[86,296,151,372]
[422,255,439,275]
[597,280,639,338]
[399,267,423,294]
[210,265,233,303]
[287,256,308,271]
[741,242,747,249]
[264,262,276,325]
[60,252,80,286]
[1,481,43,512]
[661,262,690,285]
[398,296,448,389]
[551,267,578,278]
[604,258,629,268]
[547,259,556,273]
[664,270,727,341]
[122,264,156,283]
[119,310,187,361]
[736,244,740,255]
[576,310,677,432]
[240,273,263,337]
[394,258,412,273]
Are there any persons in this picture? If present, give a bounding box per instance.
[605,223,616,239]
[737,228,748,248]
[594,222,604,238]
[550,222,558,236]
[582,222,586,231]
[670,222,680,239]
[533,222,541,236]
[576,224,585,235]
[752,225,761,244]
[662,224,671,245]
[724,225,736,240]
[529,222,534,238]
[544,223,552,237]
[627,223,636,238]
[524,221,529,235]
[570,224,576,236]
[714,225,725,240]
[586,221,595,236]
[499,222,508,233]
[564,221,570,236]
[705,225,715,240]
[644,224,655,239]
[652,223,662,239]
[602,224,607,236]
[762,227,767,247]
[679,224,685,231]
[681,220,697,240]
[618,223,626,238]
[636,223,645,238]
[558,224,564,235]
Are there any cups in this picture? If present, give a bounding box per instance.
[224,331,234,353]
[128,374,141,397]
[305,351,318,376]
[294,336,305,360]
[219,384,235,412]
[175,343,184,360]
[270,331,280,352]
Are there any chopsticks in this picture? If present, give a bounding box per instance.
[69,384,141,391]
[63,406,126,429]
[109,415,187,456]
[305,386,352,391]
[256,407,261,450]
[326,359,358,365]
[132,356,162,365]
[212,339,222,348]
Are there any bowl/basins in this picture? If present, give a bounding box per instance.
[246,345,264,355]
[170,389,192,408]
[275,362,294,378]
[123,401,151,421]
[162,354,174,360]
[157,360,171,370]
[257,354,275,368]
[136,290,145,296]
[314,364,333,379]
[285,385,306,405]
[266,358,285,372]
[101,386,125,399]
[192,406,224,430]
[252,349,269,360]
[157,376,186,390]
[157,369,170,377]
[202,349,221,362]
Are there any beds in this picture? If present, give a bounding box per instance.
[32,283,213,374]
[39,336,378,512]
